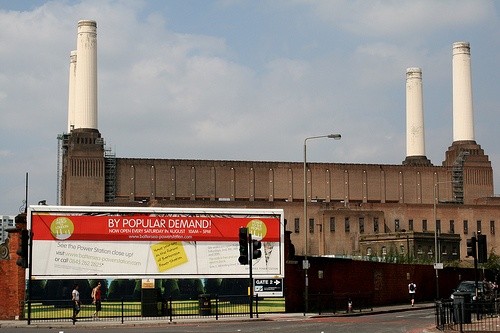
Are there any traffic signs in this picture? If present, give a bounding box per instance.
[252,278,285,298]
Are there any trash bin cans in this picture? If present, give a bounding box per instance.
[199,296,211,316]
[453,292,472,323]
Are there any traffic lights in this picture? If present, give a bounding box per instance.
[253,239,262,260]
[238,227,249,266]
[16,229,29,269]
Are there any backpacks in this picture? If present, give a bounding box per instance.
[410,285,414,291]
[91,288,95,298]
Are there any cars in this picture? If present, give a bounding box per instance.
[449,280,483,303]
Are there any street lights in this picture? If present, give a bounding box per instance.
[302,133,342,312]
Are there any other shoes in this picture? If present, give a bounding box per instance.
[72,318,78,322]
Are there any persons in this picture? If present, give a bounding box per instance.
[92,282,102,320]
[408,280,416,307]
[483,278,498,295]
[72,284,80,321]
